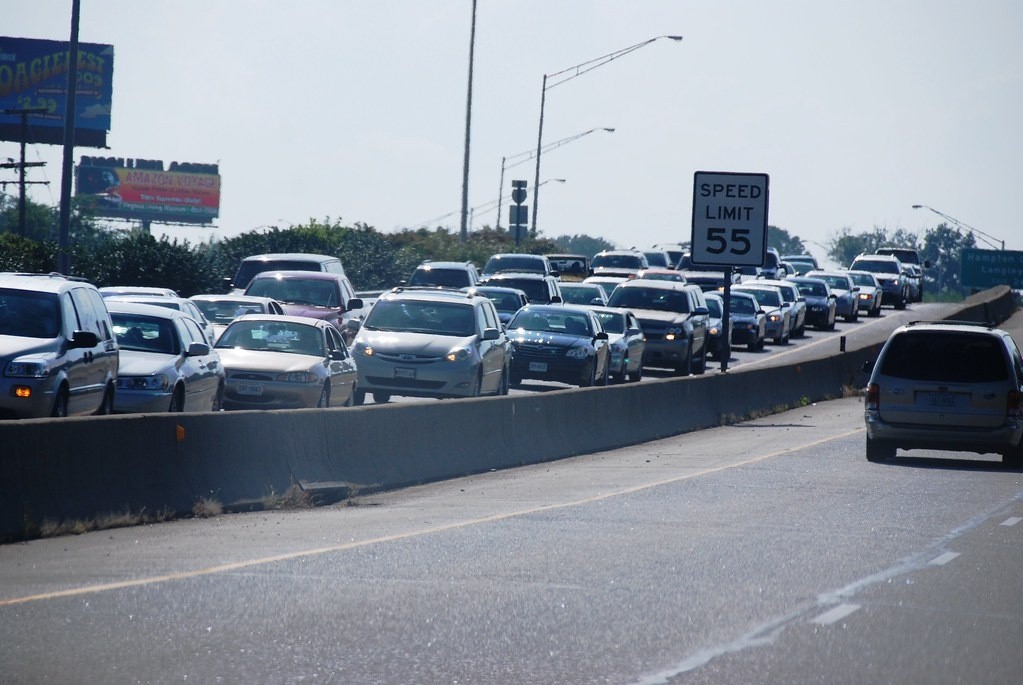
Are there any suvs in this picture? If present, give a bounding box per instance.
[861,320,1023,469]
[349,286,512,403]
[0,271,120,419]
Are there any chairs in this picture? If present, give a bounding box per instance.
[573,321,585,332]
[247,339,268,349]
[124,327,144,344]
[527,317,549,329]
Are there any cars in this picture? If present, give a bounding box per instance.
[98,253,367,412]
[399,247,931,387]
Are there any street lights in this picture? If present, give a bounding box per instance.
[912,204,1005,250]
[495,126,615,232]
[528,35,683,244]
[470,177,566,235]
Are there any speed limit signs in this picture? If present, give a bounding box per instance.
[689,171,770,268]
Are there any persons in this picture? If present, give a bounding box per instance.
[302,335,321,354]
[126,327,143,346]
[564,318,575,330]
[836,280,843,288]
[861,275,867,283]
